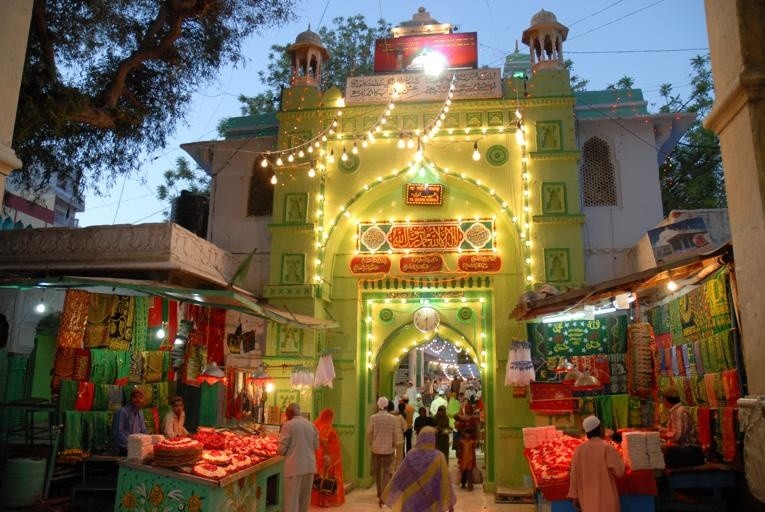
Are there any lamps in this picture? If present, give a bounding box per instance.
[554,314,573,374]
[573,307,603,391]
[563,315,583,386]
[196,306,223,382]
[246,315,273,386]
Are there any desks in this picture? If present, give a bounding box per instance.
[83,450,121,481]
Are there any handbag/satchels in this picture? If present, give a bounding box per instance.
[312,472,338,499]
[471,466,484,485]
[452,430,463,450]
[447,464,462,484]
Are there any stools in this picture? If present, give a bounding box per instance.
[68,479,117,505]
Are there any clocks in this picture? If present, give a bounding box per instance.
[413,306,440,334]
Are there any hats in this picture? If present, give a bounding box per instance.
[131,388,145,395]
[172,395,184,402]
[377,396,389,408]
[402,394,410,401]
[583,414,602,434]
[662,387,680,398]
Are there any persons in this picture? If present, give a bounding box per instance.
[275,402,318,511]
[365,373,485,512]
[109,384,148,455]
[566,415,626,512]
[655,383,704,467]
[160,396,191,440]
[310,407,346,507]
